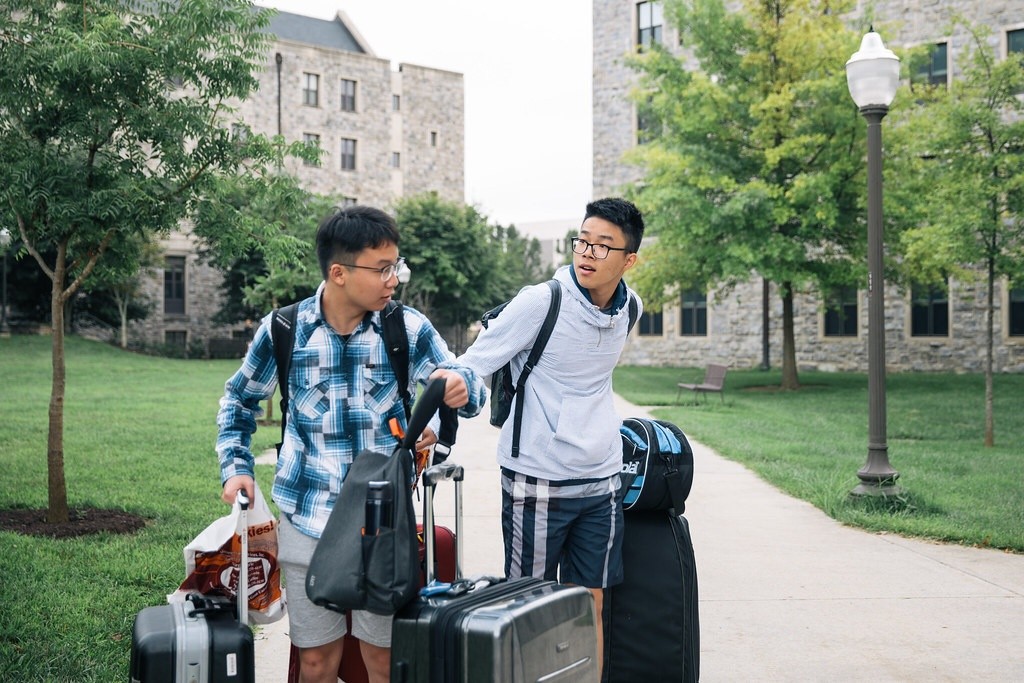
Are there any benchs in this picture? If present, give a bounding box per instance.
[674,364,728,402]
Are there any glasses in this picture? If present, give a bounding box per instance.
[571,237,632,259]
[330,257,405,282]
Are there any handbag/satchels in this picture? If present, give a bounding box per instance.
[166,481,287,624]
[304,378,458,615]
[620,417,693,518]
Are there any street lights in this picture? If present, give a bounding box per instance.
[396,262,411,306]
[0,228,12,332]
[845,26,901,452]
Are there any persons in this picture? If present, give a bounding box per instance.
[415,198,645,683]
[213,206,488,683]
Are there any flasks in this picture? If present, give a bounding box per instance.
[365,480,393,536]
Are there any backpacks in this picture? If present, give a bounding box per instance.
[481,279,638,458]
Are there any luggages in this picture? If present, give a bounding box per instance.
[602,512,700,683]
[129,489,255,683]
[288,524,457,683]
[390,463,599,683]
